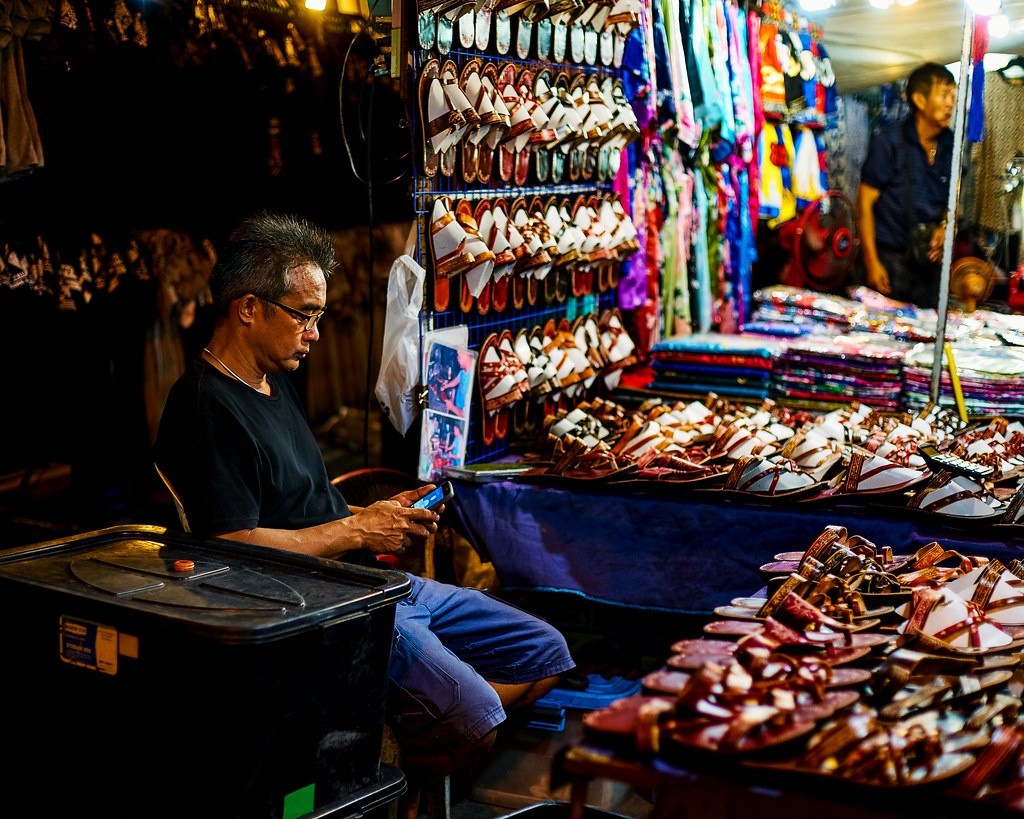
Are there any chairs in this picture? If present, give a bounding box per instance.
[152,462,436,819]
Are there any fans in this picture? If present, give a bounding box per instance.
[331,467,454,584]
[780,190,860,292]
[948,257,994,313]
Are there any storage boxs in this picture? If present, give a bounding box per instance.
[655,748,892,819]
[0,524,412,819]
[466,674,645,809]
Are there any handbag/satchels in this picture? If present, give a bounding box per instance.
[374,219,425,437]
[907,223,942,283]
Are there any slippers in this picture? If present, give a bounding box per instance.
[414,0,641,445]
[517,390,1024,528]
[580,525,1024,815]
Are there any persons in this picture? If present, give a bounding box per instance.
[157,215,576,819]
[859,60,959,309]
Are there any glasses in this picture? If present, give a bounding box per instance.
[233,290,325,331]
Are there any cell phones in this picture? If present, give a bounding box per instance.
[408,481,454,510]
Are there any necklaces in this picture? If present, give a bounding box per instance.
[204,347,265,394]
